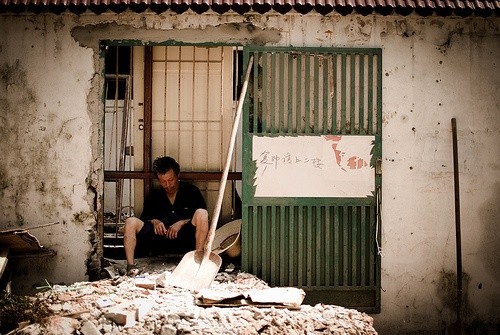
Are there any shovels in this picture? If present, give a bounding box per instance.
[168,54,254,294]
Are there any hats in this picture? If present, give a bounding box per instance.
[211,219,241,257]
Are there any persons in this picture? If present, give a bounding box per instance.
[124,156,208,276]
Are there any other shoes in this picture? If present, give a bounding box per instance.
[127,263,143,276]
